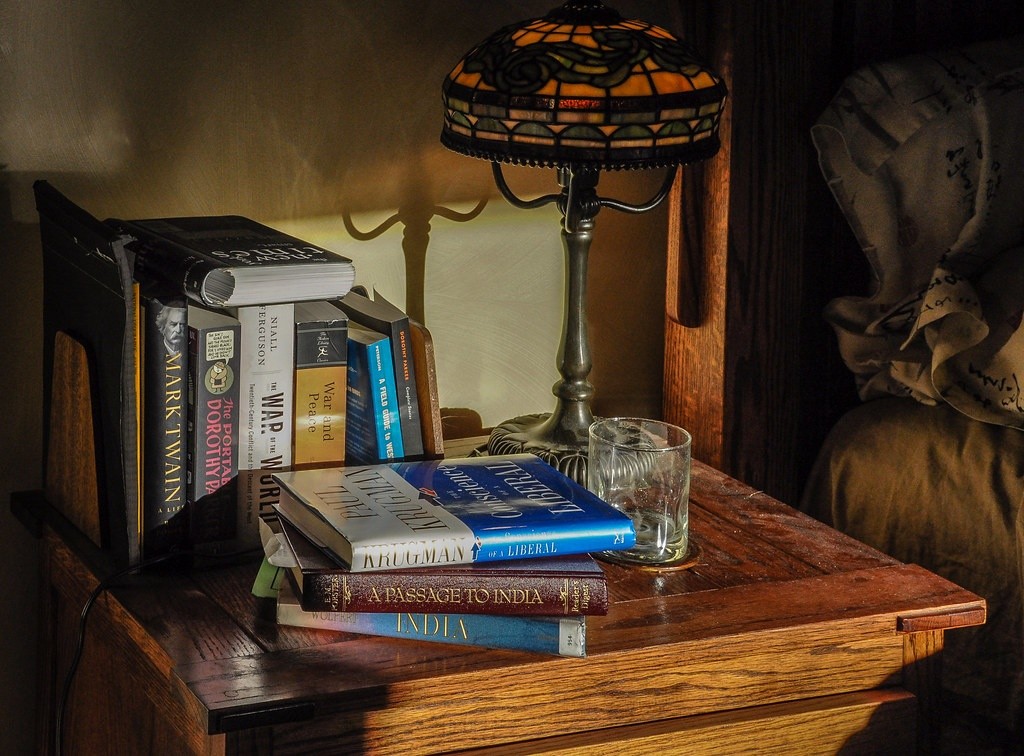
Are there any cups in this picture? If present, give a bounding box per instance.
[589,417,692,564]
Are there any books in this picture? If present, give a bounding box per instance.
[106,213,427,581]
[249,450,637,660]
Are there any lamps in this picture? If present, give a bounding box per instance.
[441,0,729,483]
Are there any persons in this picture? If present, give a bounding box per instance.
[156,307,187,364]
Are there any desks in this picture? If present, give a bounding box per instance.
[12,422,986,756]
